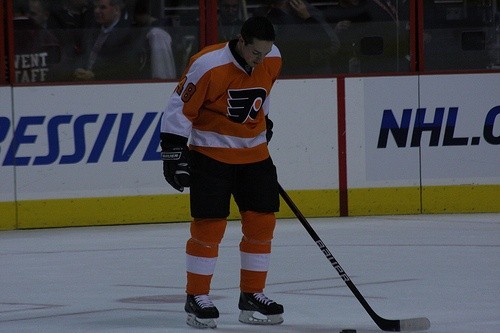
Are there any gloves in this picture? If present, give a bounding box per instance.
[158,132,194,192]
[265,112,274,146]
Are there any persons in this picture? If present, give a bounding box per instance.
[160,14,284,317]
[13,0,497,82]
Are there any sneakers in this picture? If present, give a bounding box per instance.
[237,292,285,325]
[183,292,225,331]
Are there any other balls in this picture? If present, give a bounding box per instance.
[342,329,357,333]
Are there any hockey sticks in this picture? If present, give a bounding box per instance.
[276,181,431,332]
